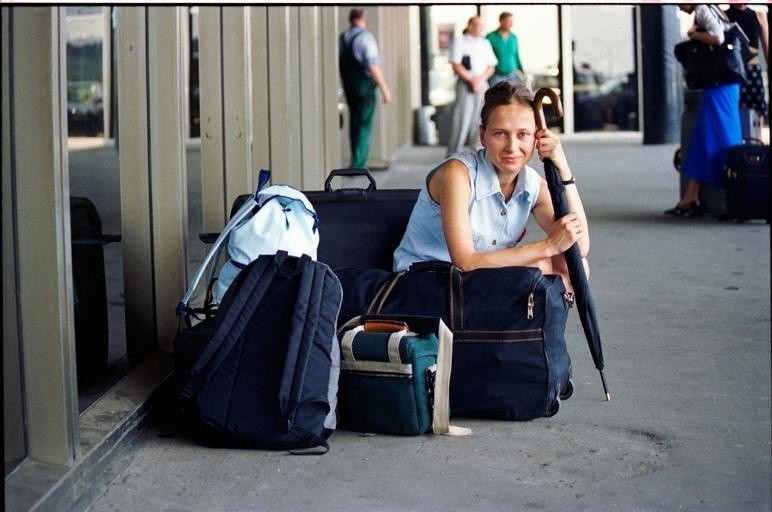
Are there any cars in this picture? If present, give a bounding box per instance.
[66,80,103,113]
[533,70,628,130]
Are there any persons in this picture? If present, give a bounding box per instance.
[558,38,585,135]
[391,80,588,401]
[664,5,743,220]
[721,4,769,144]
[446,16,499,157]
[484,12,527,89]
[339,7,390,170]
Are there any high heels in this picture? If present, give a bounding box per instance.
[663,199,702,219]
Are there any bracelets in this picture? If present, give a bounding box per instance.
[559,176,575,186]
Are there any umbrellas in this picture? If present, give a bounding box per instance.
[531,86,619,402]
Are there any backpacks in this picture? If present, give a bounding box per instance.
[179,248,345,452]
[180,184,324,324]
[339,30,369,82]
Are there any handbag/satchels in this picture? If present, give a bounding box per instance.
[333,317,454,436]
[672,29,750,90]
[325,258,575,423]
[230,166,424,270]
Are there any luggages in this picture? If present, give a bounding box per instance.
[722,138,772,224]
